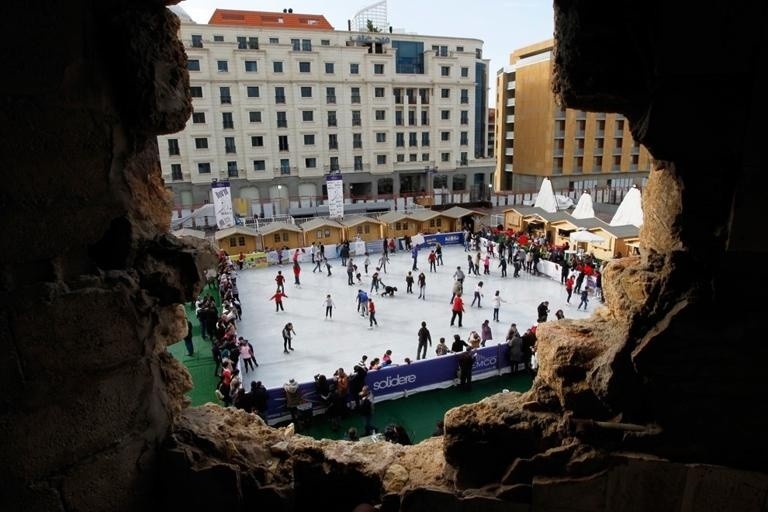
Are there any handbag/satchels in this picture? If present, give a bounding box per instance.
[215,390,224,400]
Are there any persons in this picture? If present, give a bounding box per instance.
[185,248,271,424]
[269,221,623,445]
